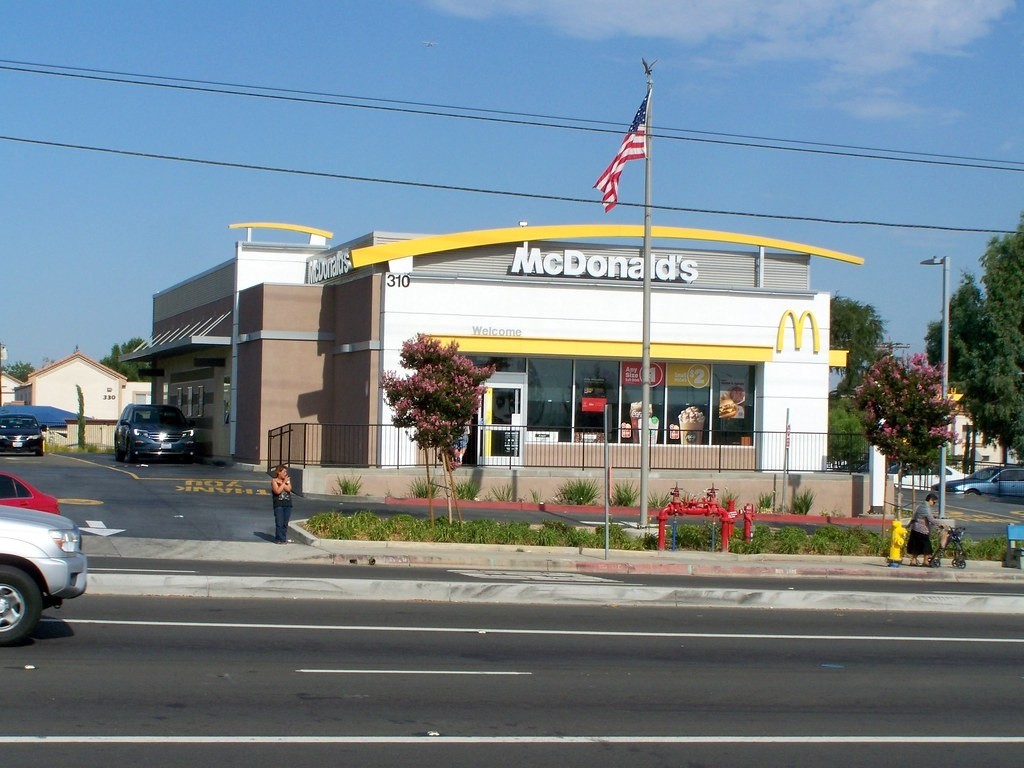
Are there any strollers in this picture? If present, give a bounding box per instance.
[927,525,967,570]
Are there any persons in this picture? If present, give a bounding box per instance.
[906,493,947,567]
[453,421,470,466]
[271,464,293,544]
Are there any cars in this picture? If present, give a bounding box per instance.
[930,466,1024,497]
[0,505,88,646]
[113,404,196,464]
[837,457,970,492]
[0,470,61,516]
[0,413,48,456]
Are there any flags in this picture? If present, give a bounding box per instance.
[591,89,653,214]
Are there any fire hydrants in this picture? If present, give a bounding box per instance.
[657,482,730,552]
[887,521,908,568]
[739,503,755,543]
[725,499,738,537]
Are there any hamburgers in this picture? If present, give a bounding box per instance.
[719,399,738,418]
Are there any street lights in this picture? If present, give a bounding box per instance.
[919,256,956,529]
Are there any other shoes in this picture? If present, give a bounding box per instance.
[910,562,923,567]
[922,563,930,566]
